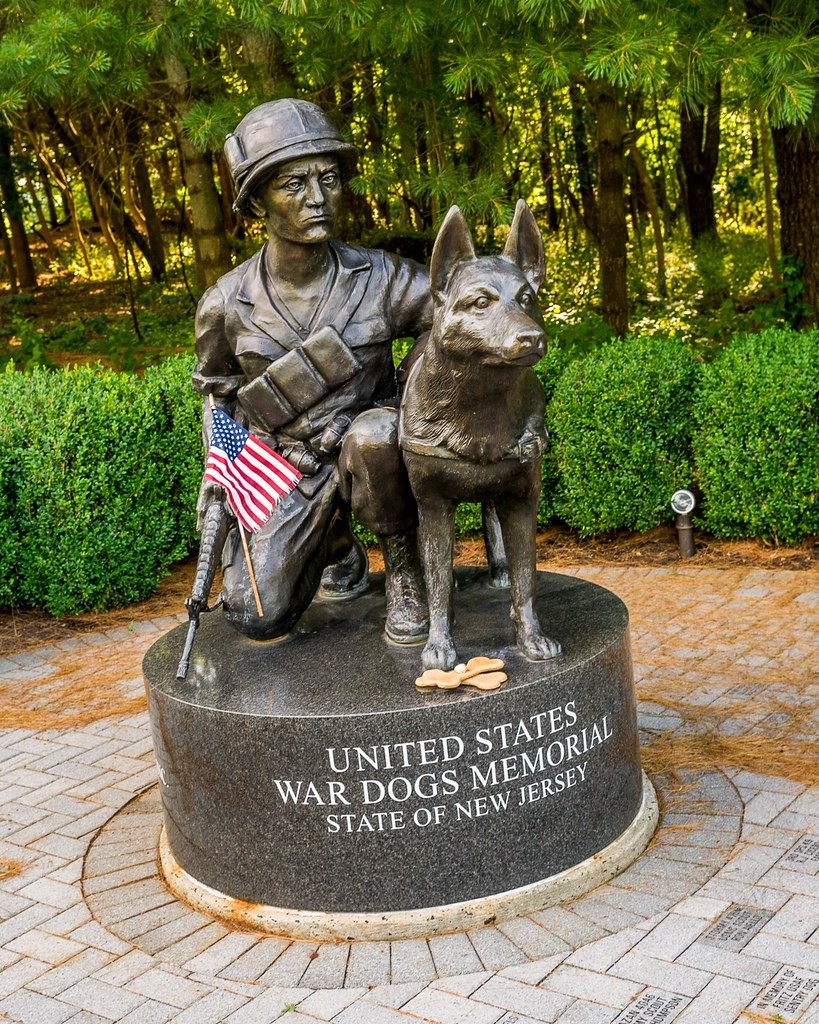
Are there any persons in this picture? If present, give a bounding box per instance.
[191,99,550,648]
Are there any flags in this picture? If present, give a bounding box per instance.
[205,407,304,535]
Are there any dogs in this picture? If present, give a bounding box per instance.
[386,196,564,671]
